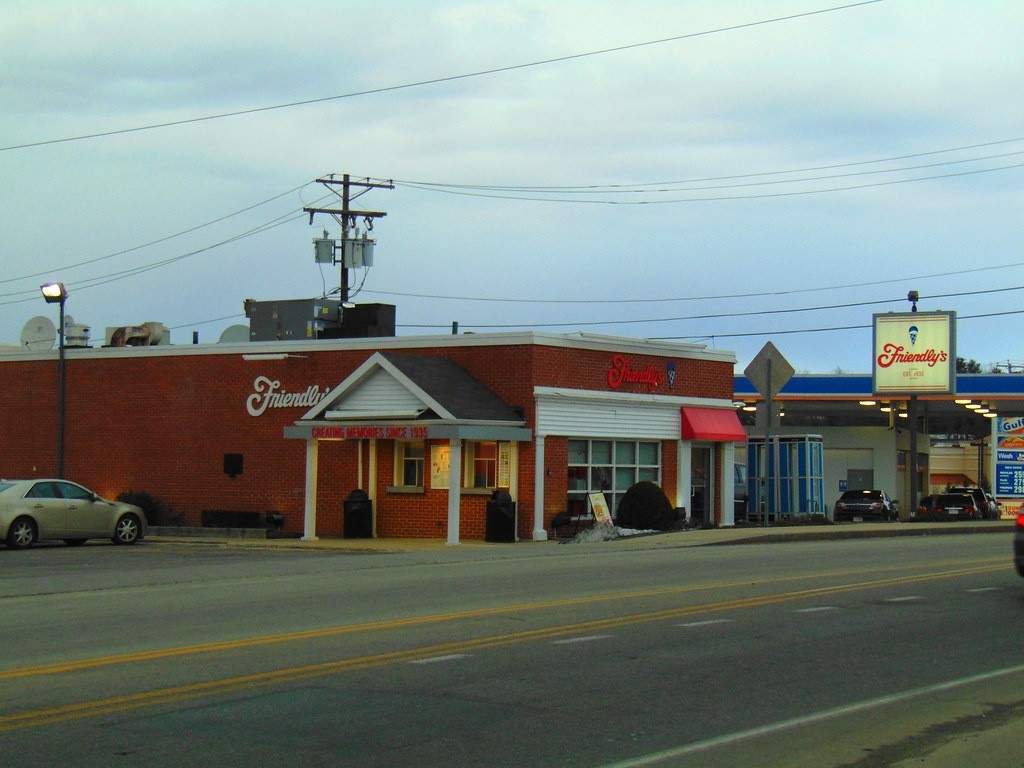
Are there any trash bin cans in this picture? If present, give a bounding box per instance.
[484,490,516,543]
[343,489,373,540]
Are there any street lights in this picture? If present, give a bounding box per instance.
[39,282,70,479]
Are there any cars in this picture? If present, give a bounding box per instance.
[0,479,149,549]
[986,493,1004,520]
[915,494,941,520]
[950,486,993,520]
[933,492,982,520]
[833,489,899,522]
